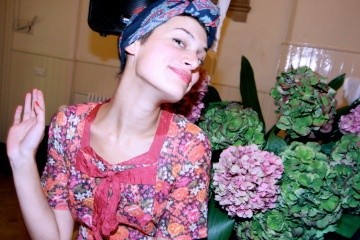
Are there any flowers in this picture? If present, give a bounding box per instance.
[161,55,360,240]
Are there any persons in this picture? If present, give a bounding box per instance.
[4,0,222,240]
